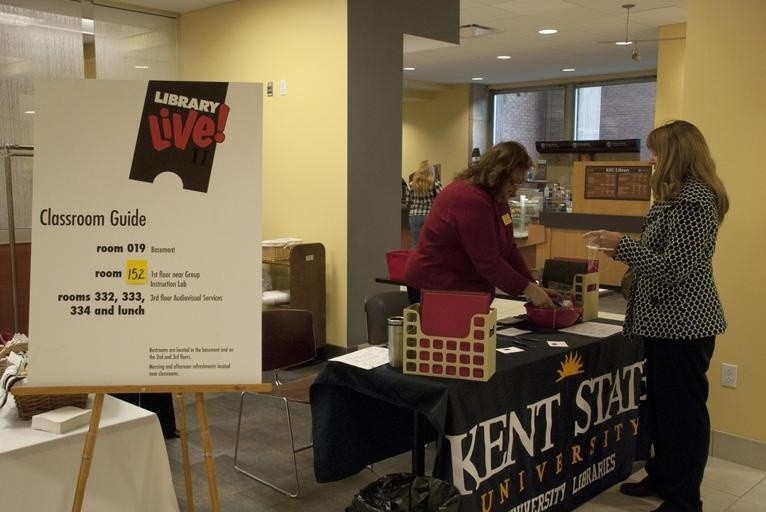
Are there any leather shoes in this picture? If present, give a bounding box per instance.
[620,473,663,498]
[650,500,702,511]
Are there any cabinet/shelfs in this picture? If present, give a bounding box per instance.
[263,241,328,354]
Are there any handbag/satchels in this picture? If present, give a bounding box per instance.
[621,266,635,299]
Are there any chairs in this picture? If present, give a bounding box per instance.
[363,290,414,348]
[226,306,376,497]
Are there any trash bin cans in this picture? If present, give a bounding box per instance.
[354,473,461,512]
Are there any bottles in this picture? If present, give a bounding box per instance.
[544,183,573,212]
[528,170,534,183]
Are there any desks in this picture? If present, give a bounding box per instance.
[304,310,654,509]
[0,366,185,511]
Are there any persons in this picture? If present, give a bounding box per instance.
[403,140,558,311]
[583,120,731,511]
[405,160,443,248]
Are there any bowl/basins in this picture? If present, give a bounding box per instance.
[521,301,582,327]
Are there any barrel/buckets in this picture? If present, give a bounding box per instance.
[384,250,412,280]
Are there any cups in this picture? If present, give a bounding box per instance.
[386,316,403,367]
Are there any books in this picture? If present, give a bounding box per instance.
[31,405,92,434]
